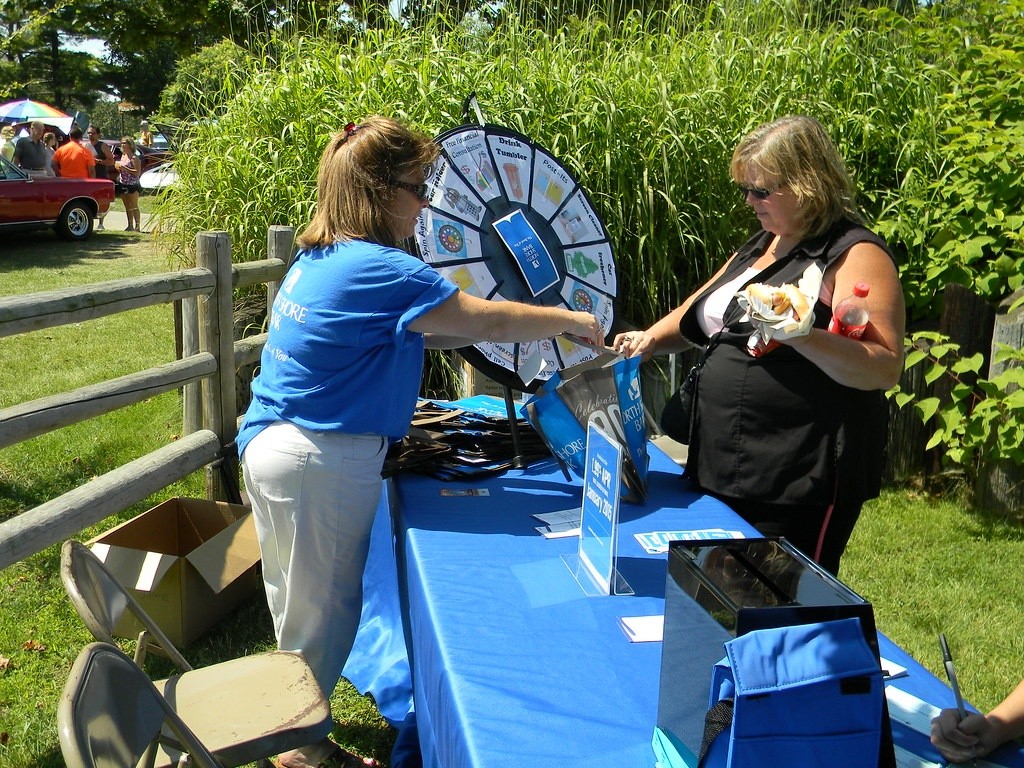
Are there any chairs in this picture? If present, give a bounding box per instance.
[56,541,332,768]
[54,641,223,767]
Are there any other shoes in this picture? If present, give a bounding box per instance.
[123,227,139,231]
[97,226,104,230]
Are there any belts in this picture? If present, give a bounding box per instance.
[23,167,44,170]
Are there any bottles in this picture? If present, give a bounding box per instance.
[827,282,869,341]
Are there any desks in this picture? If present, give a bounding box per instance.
[334,394,1022,766]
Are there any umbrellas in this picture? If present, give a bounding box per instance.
[0,98,71,124]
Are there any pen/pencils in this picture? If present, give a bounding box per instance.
[938,633,979,768]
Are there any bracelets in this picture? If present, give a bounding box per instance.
[98,159,102,165]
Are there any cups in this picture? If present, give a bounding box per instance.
[116,161,121,169]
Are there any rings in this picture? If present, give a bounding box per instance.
[595,327,601,337]
[622,336,633,342]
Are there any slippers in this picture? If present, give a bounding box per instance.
[275,746,374,767]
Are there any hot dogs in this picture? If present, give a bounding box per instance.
[745,281,809,324]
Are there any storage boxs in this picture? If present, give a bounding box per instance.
[88,495,260,649]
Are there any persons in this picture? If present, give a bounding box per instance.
[116,136,141,232]
[13,121,55,178]
[139,120,153,148]
[43,133,56,154]
[51,127,95,178]
[0,126,18,180]
[932,676,1023,762]
[236,110,603,768]
[609,115,906,581]
[82,124,115,231]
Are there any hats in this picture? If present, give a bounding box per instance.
[138,120,148,126]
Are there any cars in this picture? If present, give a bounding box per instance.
[1,157,116,241]
[100,124,189,193]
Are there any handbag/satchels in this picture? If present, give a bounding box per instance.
[660,369,700,446]
[520,330,651,504]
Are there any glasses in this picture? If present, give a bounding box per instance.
[384,176,428,199]
[735,179,786,200]
